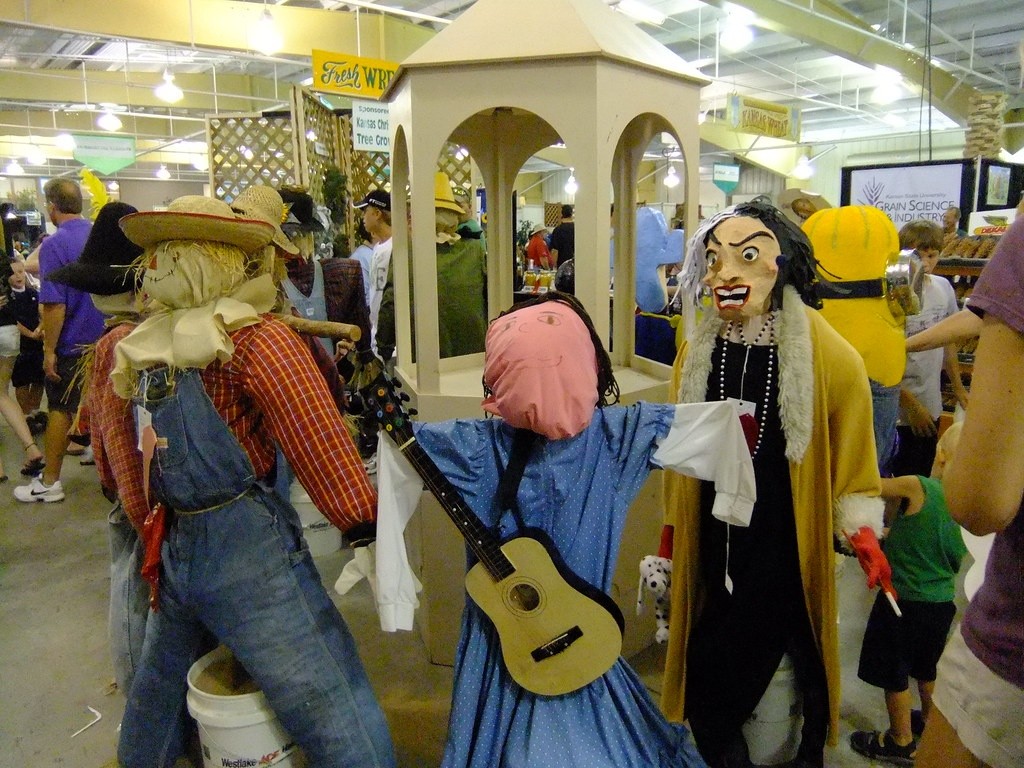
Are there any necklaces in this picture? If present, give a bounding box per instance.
[738,314,773,346]
[718,314,775,461]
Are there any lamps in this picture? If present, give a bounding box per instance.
[664,161,679,188]
[564,168,577,195]
[156,43,184,103]
[156,140,171,179]
[6,135,24,176]
[795,148,813,180]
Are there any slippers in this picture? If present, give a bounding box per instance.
[0,476,9,482]
[65,448,85,455]
[20,462,46,476]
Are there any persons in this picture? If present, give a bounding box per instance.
[892,220,971,513]
[112,181,379,768]
[661,197,884,768]
[372,170,490,356]
[939,205,965,236]
[849,415,965,768]
[0,180,392,502]
[45,197,201,767]
[909,198,1024,768]
[523,205,575,270]
[379,288,757,768]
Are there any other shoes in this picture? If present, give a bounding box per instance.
[25,409,48,435]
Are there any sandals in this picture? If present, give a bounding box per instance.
[910,708,926,737]
[850,730,917,765]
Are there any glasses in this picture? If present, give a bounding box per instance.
[451,187,470,197]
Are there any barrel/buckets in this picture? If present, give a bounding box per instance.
[186,645,310,768]
[741,652,804,766]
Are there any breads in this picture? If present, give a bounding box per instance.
[940,232,1000,406]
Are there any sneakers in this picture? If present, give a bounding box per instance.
[14,474,65,503]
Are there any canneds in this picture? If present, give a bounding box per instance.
[524,270,556,287]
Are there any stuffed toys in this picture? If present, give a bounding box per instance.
[636,555,673,643]
[799,205,924,580]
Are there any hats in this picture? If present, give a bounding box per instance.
[229,185,299,255]
[407,172,466,215]
[351,189,391,211]
[531,223,547,235]
[276,189,325,233]
[41,170,146,295]
[118,195,274,256]
[452,185,469,204]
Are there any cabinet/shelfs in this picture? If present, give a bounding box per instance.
[932,234,1002,413]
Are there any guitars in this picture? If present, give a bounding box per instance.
[358,372,626,696]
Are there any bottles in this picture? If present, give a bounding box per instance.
[667,274,682,313]
[516,262,557,293]
